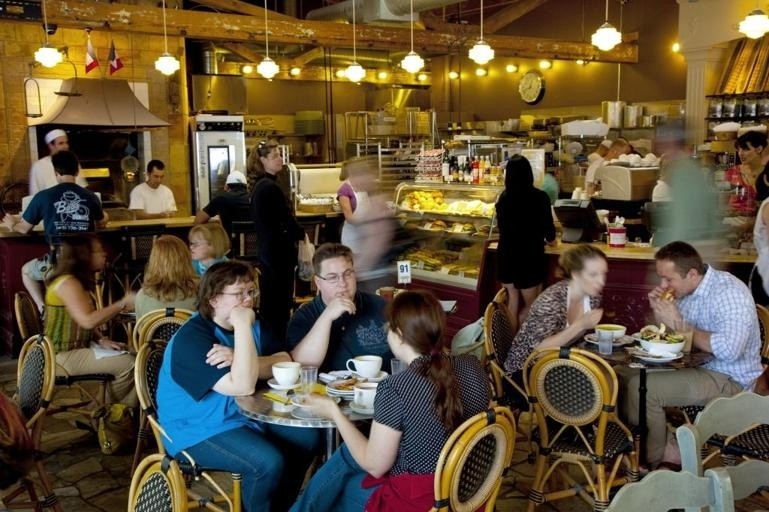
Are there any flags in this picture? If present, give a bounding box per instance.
[108,40,124,75]
[84,37,99,73]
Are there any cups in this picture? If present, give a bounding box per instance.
[380,286,395,301]
[346,356,383,378]
[354,382,378,409]
[673,319,697,355]
[272,391,293,413]
[300,365,317,386]
[598,330,613,354]
[272,362,301,386]
[391,357,404,376]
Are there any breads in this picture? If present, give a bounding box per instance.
[449,267,478,278]
[479,223,489,236]
[419,220,446,234]
[406,252,441,271]
[449,223,474,236]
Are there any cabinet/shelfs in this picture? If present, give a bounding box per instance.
[704,92,769,157]
[348,140,425,182]
[391,180,505,337]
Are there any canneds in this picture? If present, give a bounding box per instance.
[609,227,626,247]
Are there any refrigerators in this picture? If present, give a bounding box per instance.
[191,116,247,214]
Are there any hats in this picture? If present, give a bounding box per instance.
[226,171,247,184]
[45,129,66,144]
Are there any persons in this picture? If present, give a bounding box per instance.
[618,137,644,159]
[190,224,232,276]
[726,130,767,245]
[624,241,763,476]
[154,262,317,512]
[587,140,611,167]
[43,233,139,424]
[286,243,395,371]
[195,171,257,261]
[750,163,769,306]
[288,291,491,512]
[248,139,304,354]
[501,244,648,476]
[337,161,398,269]
[136,235,201,333]
[128,159,178,218]
[543,154,558,203]
[495,155,556,333]
[584,138,629,195]
[4,151,103,321]
[644,119,722,325]
[30,129,90,193]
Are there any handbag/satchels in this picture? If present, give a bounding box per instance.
[451,317,485,360]
[532,416,598,449]
[97,404,133,456]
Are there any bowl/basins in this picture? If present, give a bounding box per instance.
[595,324,626,337]
[640,338,685,353]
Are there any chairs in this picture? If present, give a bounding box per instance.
[129,233,159,261]
[1,268,769,512]
[229,219,260,258]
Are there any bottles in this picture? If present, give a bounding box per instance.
[609,228,627,248]
[442,155,503,186]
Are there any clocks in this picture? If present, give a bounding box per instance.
[518,69,546,105]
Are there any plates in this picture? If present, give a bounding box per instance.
[290,407,324,420]
[267,378,303,390]
[584,333,634,347]
[632,333,640,342]
[327,381,355,400]
[119,312,136,317]
[290,395,342,408]
[632,351,684,363]
[368,371,388,382]
[349,401,374,415]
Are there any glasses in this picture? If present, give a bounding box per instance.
[315,270,354,282]
[190,240,209,246]
[220,288,256,297]
[258,141,266,150]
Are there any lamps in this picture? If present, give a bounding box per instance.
[467,0,495,65]
[738,0,769,40]
[375,71,387,80]
[238,64,253,74]
[539,60,552,70]
[154,0,181,77]
[414,74,427,83]
[32,0,64,68]
[287,66,301,77]
[576,60,589,65]
[590,0,623,53]
[447,71,460,80]
[256,1,280,80]
[399,0,425,74]
[505,64,519,73]
[475,67,488,77]
[333,69,345,79]
[343,0,366,83]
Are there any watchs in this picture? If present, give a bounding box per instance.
[118,300,125,310]
